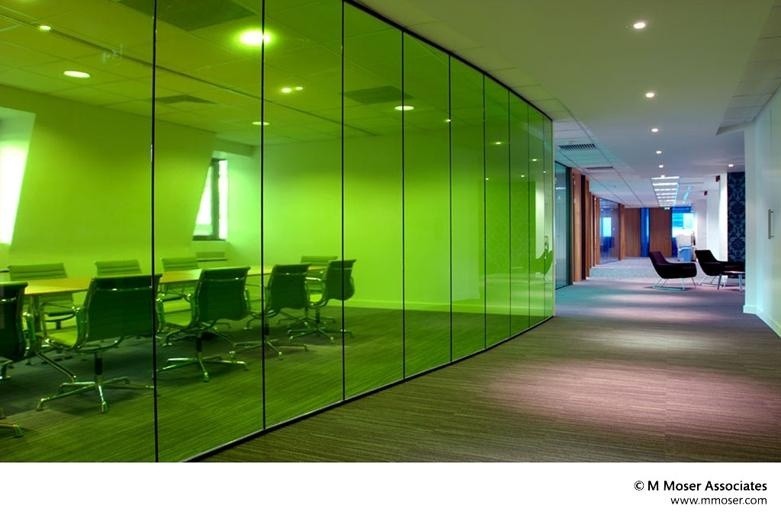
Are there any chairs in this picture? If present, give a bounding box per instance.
[647,250,744,292]
